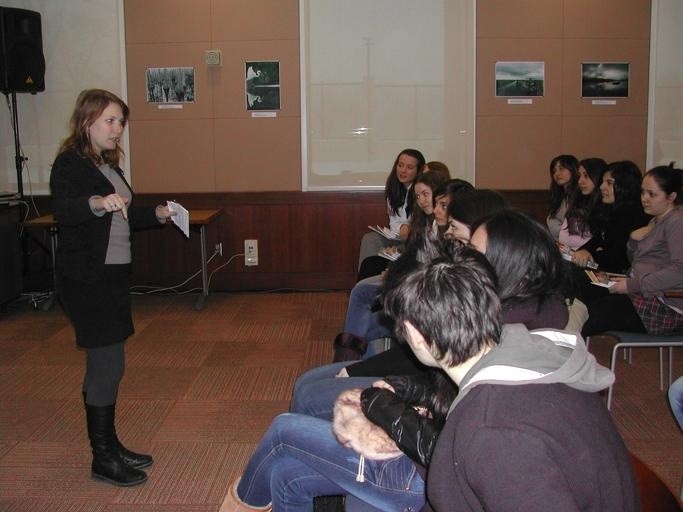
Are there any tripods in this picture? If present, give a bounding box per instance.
[11,93,54,289]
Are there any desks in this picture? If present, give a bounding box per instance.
[27,210,222,311]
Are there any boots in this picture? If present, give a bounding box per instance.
[84,402,147,486]
[82,391,153,469]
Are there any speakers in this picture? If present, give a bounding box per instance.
[0,6,45,93]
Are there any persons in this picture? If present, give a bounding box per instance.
[385,238,640,512]
[163,84,172,102]
[545,154,683,339]
[49,88,179,488]
[221,211,569,512]
[291,149,512,420]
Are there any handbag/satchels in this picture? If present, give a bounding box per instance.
[333,387,427,462]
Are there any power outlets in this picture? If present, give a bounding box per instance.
[244,239,259,266]
[216,242,222,255]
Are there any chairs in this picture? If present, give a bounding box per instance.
[586,291,683,411]
[629,452,683,512]
